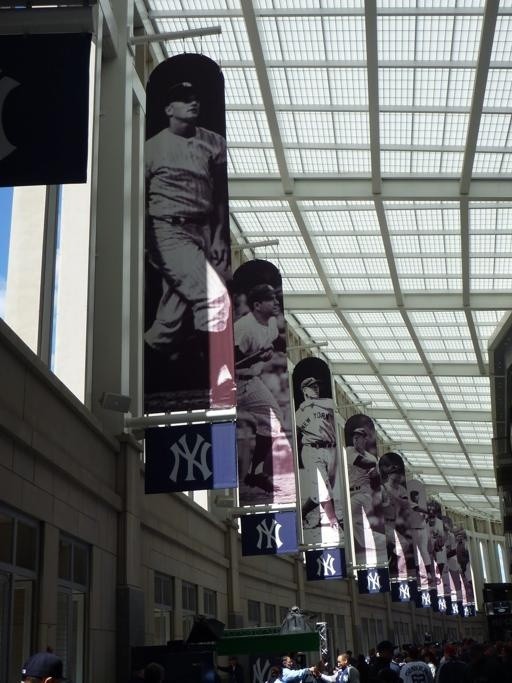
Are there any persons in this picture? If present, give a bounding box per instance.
[383,457,420,583]
[408,491,476,605]
[345,426,386,554]
[296,374,345,550]
[143,77,240,405]
[237,284,288,495]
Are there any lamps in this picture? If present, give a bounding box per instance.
[95,392,133,413]
[214,494,235,508]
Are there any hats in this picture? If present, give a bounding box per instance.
[246,284,282,301]
[21,651,67,680]
[163,81,202,107]
[377,640,398,650]
[351,427,367,437]
[301,377,322,388]
[387,465,401,474]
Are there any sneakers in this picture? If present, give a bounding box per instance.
[302,518,316,529]
[244,472,281,493]
[330,521,344,539]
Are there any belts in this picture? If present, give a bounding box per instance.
[311,443,336,448]
[386,518,395,520]
[350,486,360,491]
[172,216,195,224]
[238,374,254,381]
